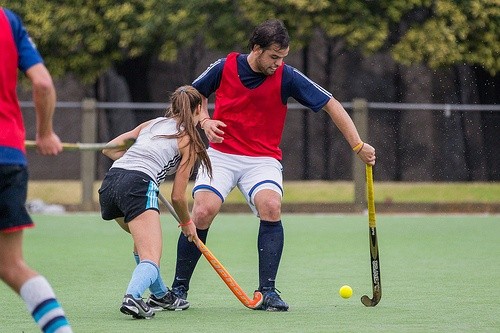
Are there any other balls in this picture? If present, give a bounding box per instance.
[339,285,353,298]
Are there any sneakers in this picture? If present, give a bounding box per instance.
[254,286,289,312]
[120,294,155,319]
[146,287,190,312]
[170,286,188,300]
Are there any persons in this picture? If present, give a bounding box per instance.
[98,85,214,319]
[170,17,376,313]
[0,4,73,333]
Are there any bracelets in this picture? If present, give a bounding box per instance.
[179,220,192,226]
[199,117,210,129]
[352,141,364,154]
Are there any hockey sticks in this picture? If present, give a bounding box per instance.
[25,137,136,151]
[360,163,383,307]
[158,191,263,309]
